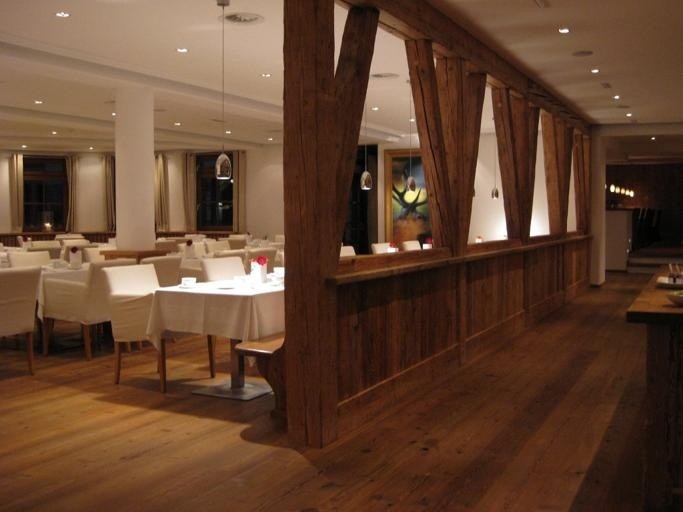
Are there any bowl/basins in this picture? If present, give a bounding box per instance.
[666,291,682,308]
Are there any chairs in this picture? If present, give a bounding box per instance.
[0,234,288,401]
[630,204,664,252]
[340,237,433,258]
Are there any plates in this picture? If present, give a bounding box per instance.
[657,276,683,287]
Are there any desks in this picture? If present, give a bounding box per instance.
[620,261,682,512]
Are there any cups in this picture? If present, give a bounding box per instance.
[273,267,284,278]
[181,277,195,288]
[261,240,268,248]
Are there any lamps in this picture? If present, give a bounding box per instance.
[360,97,373,192]
[491,146,501,201]
[214,0,233,180]
[406,78,418,193]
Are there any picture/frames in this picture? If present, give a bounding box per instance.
[383,148,434,247]
